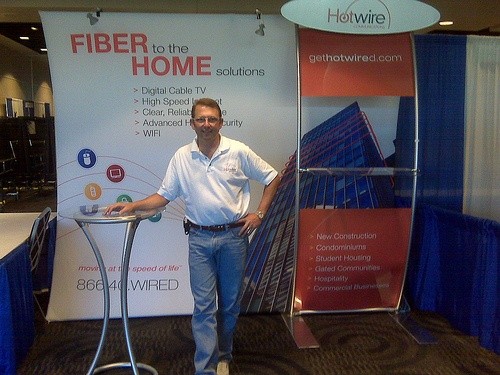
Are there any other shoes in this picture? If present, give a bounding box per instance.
[216,358,230,375]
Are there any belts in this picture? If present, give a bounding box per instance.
[190,222,245,231]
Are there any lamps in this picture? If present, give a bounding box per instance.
[255,9,265,37]
[86,7,102,25]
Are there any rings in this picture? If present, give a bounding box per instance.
[247,227,250,233]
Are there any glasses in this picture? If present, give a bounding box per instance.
[193,116,221,124]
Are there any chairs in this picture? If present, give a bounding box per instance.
[29,207,51,322]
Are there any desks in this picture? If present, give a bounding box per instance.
[58,204,166,375]
[0,212,56,375]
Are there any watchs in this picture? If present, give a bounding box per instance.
[256,210,264,219]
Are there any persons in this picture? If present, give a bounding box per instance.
[104,98,280,375]
